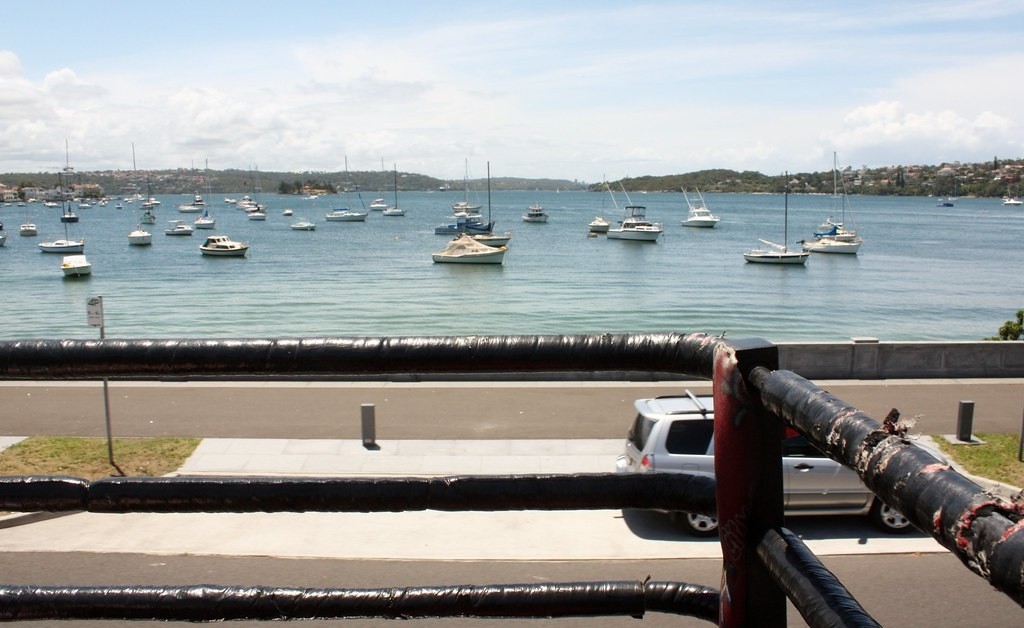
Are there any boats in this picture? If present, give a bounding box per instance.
[1002,197,1024,207]
[59,253,93,276]
[430,155,511,265]
[18,223,37,236]
[290,220,317,231]
[936,201,955,208]
[678,187,722,228]
[0,232,8,246]
[588,217,610,233]
[604,178,663,241]
[283,208,294,218]
[200,236,251,256]
[4,202,12,206]
[369,199,389,211]
[521,201,548,224]
[18,201,26,207]
[27,198,37,203]
[164,220,195,237]
[303,195,319,201]
[43,173,161,224]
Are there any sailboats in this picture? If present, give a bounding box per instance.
[792,150,863,255]
[176,154,268,229]
[36,171,85,252]
[743,169,812,264]
[127,140,154,246]
[325,153,369,222]
[382,161,405,216]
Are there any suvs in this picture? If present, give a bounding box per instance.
[619,391,951,537]
